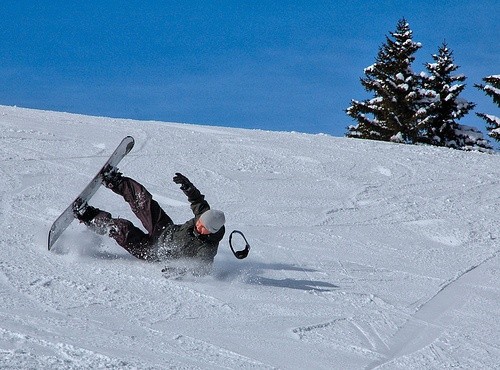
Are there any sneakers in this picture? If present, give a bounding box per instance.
[72,198,89,224]
[101,164,124,188]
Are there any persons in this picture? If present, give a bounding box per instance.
[74,164,225,280]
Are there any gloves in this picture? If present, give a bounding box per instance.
[160,267,187,280]
[173,173,193,190]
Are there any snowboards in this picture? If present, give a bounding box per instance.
[48,136,134,252]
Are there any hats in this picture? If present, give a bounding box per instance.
[200,210,225,233]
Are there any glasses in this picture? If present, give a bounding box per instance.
[229,230,250,259]
